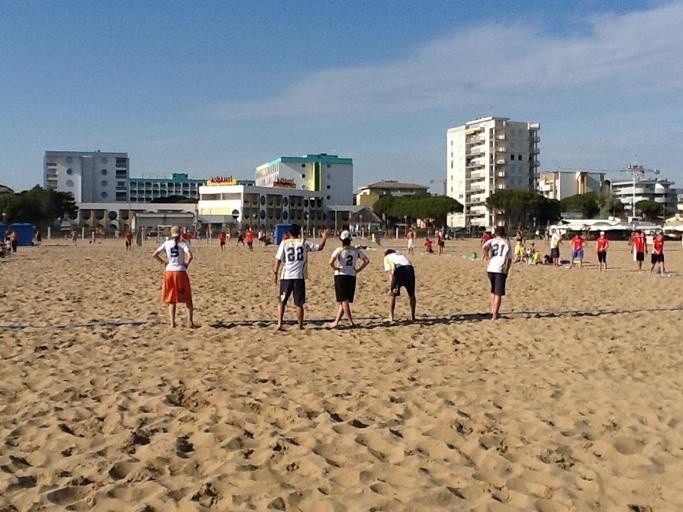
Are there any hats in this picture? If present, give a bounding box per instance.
[340,230,352,241]
[171,226,179,238]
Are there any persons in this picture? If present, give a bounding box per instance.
[482,226,512,320]
[383,249,417,323]
[480,225,665,275]
[71,229,78,246]
[125,231,133,251]
[328,230,369,328]
[218,226,267,251]
[406,227,446,255]
[272,223,330,331]
[4,229,18,254]
[152,225,198,329]
[35,230,42,248]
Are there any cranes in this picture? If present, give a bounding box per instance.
[428,176,446,194]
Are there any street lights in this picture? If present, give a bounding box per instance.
[359,214,362,231]
[303,196,315,231]
[626,163,644,217]
[252,213,257,231]
[1,212,6,223]
[403,215,407,235]
